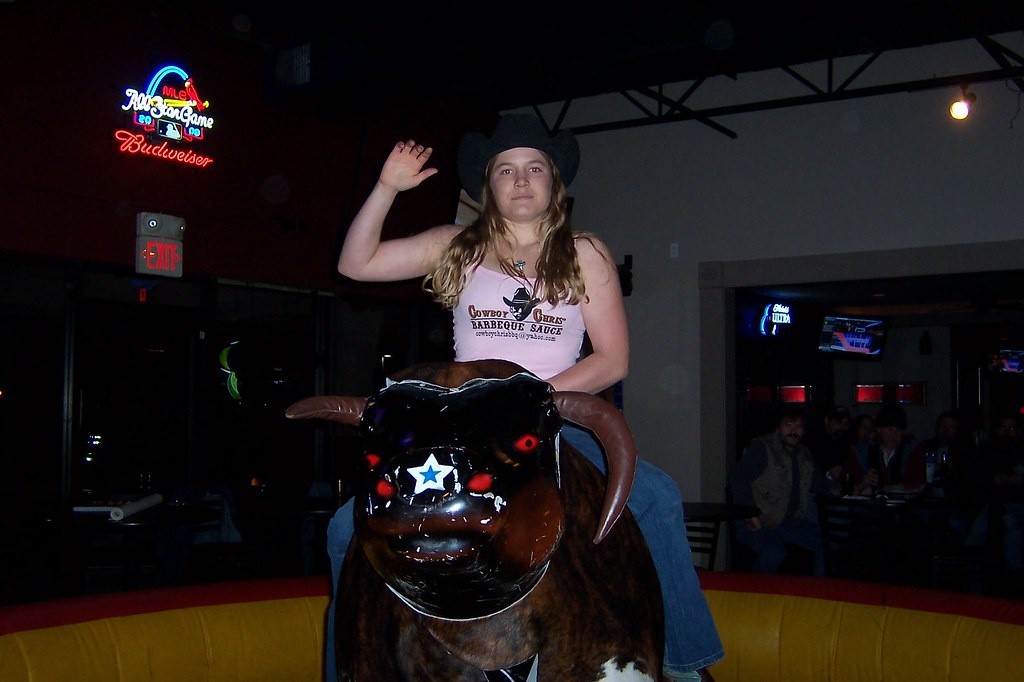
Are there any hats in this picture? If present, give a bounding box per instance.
[876,406,908,429]
[456,115,581,186]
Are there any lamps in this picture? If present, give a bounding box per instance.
[948,80,979,121]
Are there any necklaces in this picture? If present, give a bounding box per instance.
[496,232,540,275]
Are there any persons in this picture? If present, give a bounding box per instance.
[322,115,724,682]
[724,388,1024,600]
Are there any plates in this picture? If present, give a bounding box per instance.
[880,491,920,499]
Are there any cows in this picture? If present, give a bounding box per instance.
[286,358,717,682]
